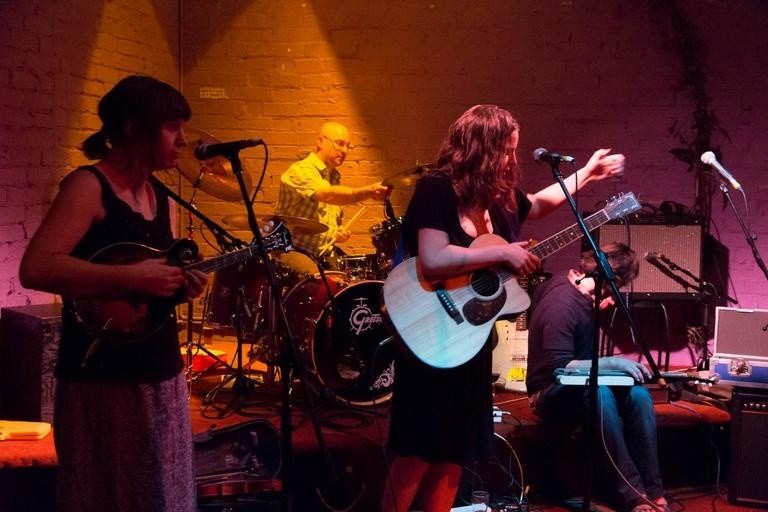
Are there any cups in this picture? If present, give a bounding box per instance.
[472,490,491,511]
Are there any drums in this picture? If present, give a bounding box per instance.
[273,270,395,406]
[368,215,409,271]
[338,253,385,280]
[207,256,298,337]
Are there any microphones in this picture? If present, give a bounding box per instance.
[530,145,577,170]
[701,149,743,193]
[192,136,264,161]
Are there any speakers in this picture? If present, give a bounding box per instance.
[0,302,62,423]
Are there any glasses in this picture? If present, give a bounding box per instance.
[322,136,354,150]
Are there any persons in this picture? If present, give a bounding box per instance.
[18,74,206,512]
[527,243,672,512]
[375,104,628,510]
[267,123,393,406]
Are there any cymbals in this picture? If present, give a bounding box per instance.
[174,127,253,202]
[382,162,434,190]
[223,213,329,235]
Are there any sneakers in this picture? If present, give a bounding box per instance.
[632,496,669,512]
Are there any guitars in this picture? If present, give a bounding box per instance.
[382,191,642,373]
[493,240,543,393]
[69,219,292,344]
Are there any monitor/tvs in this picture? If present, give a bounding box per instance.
[713,303,768,365]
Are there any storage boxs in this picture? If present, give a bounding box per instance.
[708,307,768,389]
